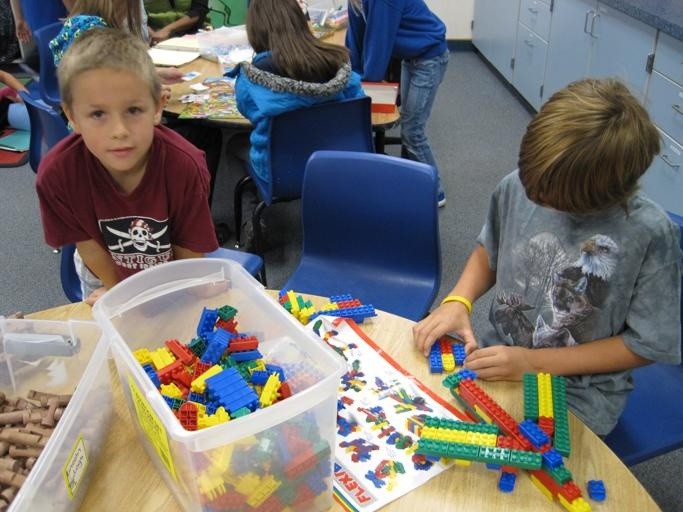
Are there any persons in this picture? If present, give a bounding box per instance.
[48,0,148,134]
[0,70,29,132]
[36,25,230,307]
[9,0,79,49]
[412,79,681,445]
[143,0,210,47]
[223,1,367,252]
[344,1,450,209]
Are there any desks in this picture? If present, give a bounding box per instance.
[148,24,400,215]
[0,289,662,512]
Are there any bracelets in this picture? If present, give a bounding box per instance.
[440,296,472,314]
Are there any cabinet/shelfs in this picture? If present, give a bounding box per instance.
[637,30,683,212]
[513,0,552,111]
[541,1,657,109]
[471,0,520,82]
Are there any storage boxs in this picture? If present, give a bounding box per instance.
[89,256,351,512]
[0,314,114,511]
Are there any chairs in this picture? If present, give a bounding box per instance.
[33,22,62,104]
[58,233,268,303]
[594,210,683,465]
[19,91,68,174]
[229,98,375,285]
[279,150,442,321]
